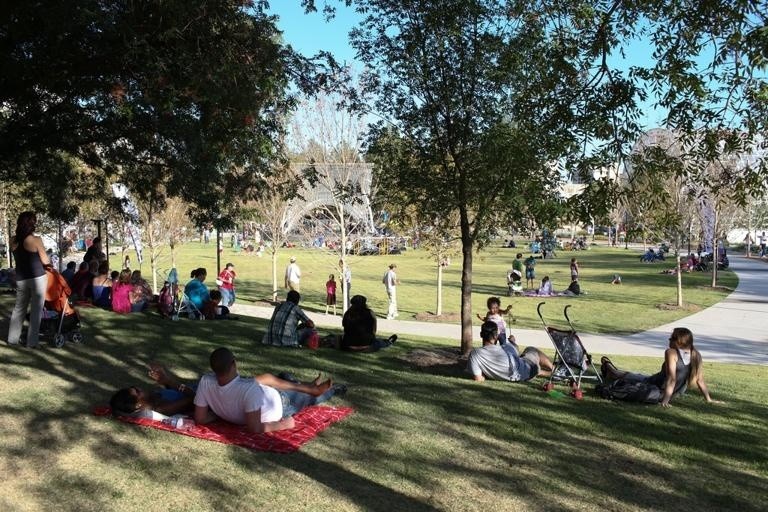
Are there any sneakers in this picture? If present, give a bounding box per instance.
[389,335,397,343]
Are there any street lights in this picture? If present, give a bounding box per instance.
[212,208,222,281]
[103,213,113,269]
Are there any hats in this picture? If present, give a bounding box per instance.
[351,295,366,304]
[226,263,233,267]
[290,256,296,262]
[388,264,397,267]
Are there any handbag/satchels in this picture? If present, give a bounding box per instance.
[604,379,661,404]
[159,280,173,315]
[215,280,223,286]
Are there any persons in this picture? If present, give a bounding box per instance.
[108,359,333,417]
[339,293,398,353]
[189,346,348,435]
[476,297,512,348]
[334,259,352,309]
[217,220,423,256]
[65,235,240,321]
[324,274,337,316]
[757,231,768,258]
[284,255,302,293]
[743,233,756,254]
[601,326,723,407]
[381,263,400,321]
[499,221,730,297]
[261,290,318,348]
[6,211,52,349]
[467,320,569,383]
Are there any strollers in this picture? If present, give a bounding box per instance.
[154,268,205,322]
[535,301,613,402]
[505,269,525,298]
[22,263,82,347]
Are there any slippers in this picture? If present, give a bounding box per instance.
[601,357,617,377]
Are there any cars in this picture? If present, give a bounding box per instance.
[556,224,628,242]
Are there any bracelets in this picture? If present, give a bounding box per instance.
[177,383,187,392]
